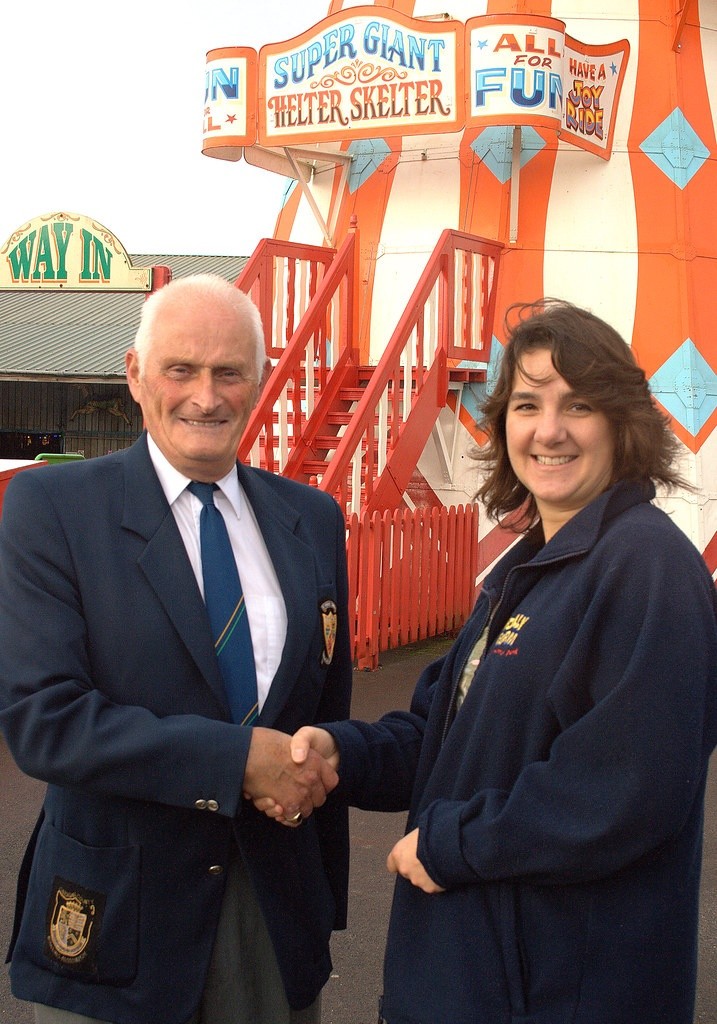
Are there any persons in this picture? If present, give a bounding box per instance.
[240,294,715,1024]
[2,271,354,1022]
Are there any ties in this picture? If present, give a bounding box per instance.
[187,480,259,728]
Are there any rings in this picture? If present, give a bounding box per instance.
[284,811,303,826]
